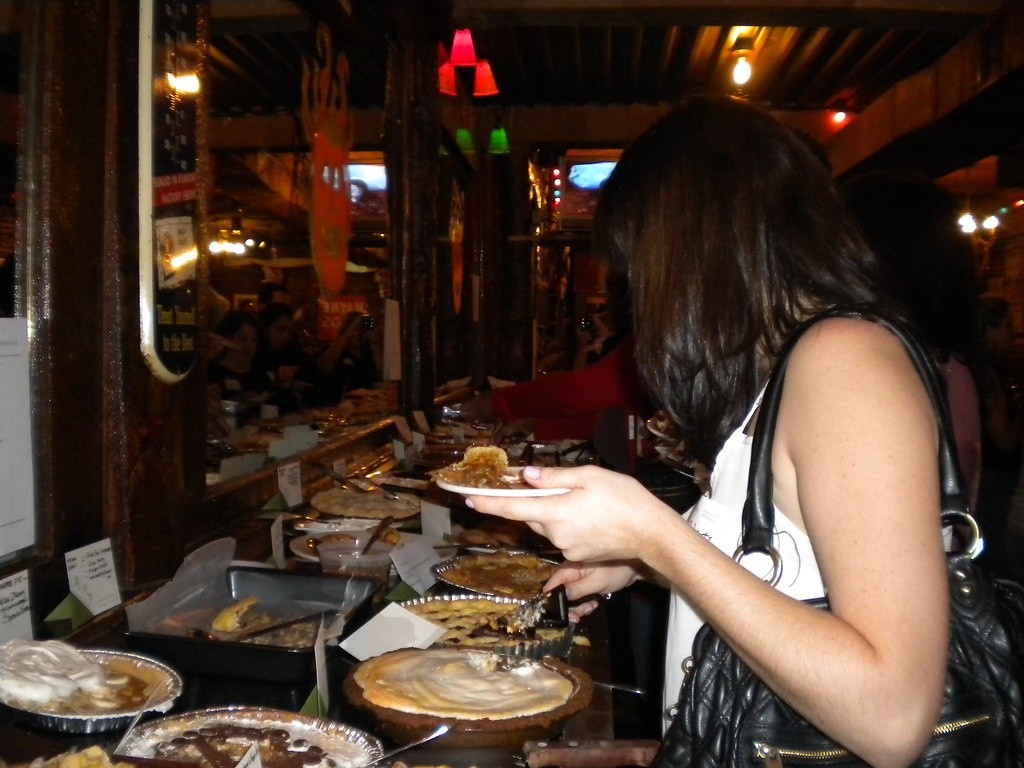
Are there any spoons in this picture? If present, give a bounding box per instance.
[187,608,340,644]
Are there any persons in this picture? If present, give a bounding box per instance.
[257,300,325,407]
[460,99,977,768]
[585,311,621,363]
[306,310,377,406]
[927,338,982,519]
[954,296,1024,580]
[204,330,241,362]
[202,309,276,397]
[462,257,709,712]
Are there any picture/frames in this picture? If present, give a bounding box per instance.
[422,118,485,412]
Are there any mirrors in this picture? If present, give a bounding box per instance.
[179,0,404,543]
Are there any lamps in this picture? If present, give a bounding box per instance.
[450,22,500,99]
[454,114,475,154]
[487,111,509,156]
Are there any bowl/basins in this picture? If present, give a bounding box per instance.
[313,540,391,606]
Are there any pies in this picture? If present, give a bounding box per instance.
[311,487,421,520]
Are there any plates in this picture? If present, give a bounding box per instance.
[430,466,572,499]
[290,532,362,560]
[452,542,563,562]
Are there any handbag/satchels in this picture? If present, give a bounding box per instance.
[650,302,1024,768]
